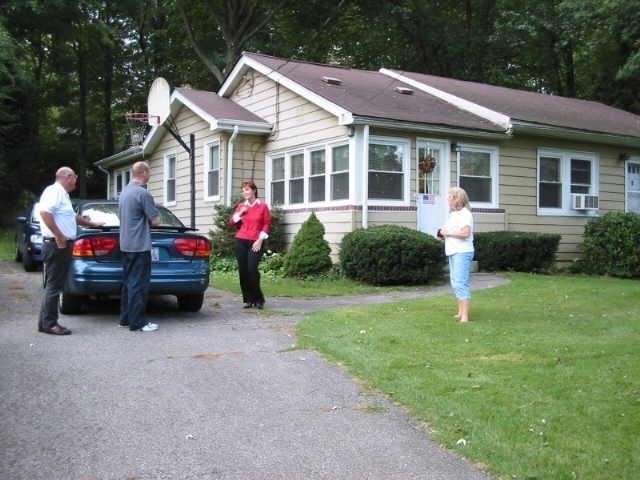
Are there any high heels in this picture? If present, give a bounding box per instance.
[243,302,256,308]
[257,302,263,309]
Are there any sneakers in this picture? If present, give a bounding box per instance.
[136,321,160,331]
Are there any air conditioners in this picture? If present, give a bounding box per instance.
[570,193,600,211]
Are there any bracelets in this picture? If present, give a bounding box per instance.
[445,231,449,237]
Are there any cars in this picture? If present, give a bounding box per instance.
[42,199,211,314]
[16,201,80,272]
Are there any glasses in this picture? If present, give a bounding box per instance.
[68,175,78,179]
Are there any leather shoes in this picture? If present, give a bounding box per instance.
[41,323,73,334]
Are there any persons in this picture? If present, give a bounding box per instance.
[34,165,105,336]
[229,181,272,310]
[437,186,475,323]
[118,159,162,333]
